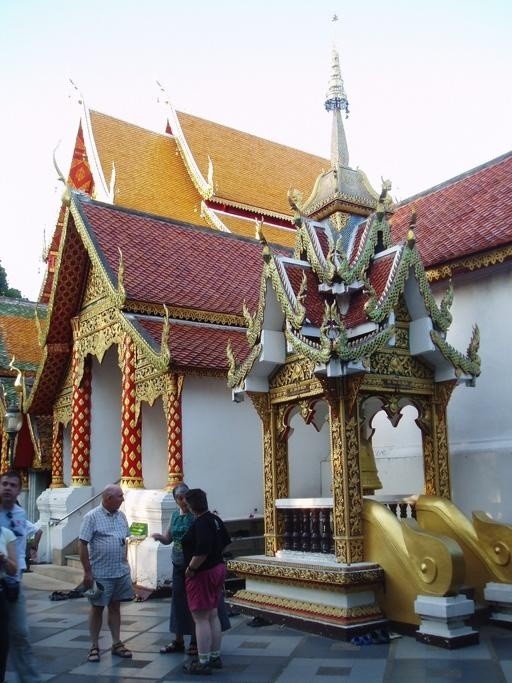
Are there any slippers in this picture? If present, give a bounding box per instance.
[351,627,402,645]
[247,614,272,626]
[224,603,241,615]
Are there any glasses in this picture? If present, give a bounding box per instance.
[6,510,15,528]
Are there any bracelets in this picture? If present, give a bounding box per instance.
[188,565,195,572]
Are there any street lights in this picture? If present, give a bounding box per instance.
[3,394,23,473]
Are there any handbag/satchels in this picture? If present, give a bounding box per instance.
[2,576,19,602]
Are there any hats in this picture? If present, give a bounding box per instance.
[79,580,104,599]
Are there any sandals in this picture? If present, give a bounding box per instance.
[88,648,99,662]
[183,656,223,674]
[112,643,132,658]
[160,640,185,653]
[187,642,198,656]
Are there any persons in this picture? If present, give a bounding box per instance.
[180,489,232,675]
[151,483,199,656]
[78,484,132,662]
[0,471,43,683]
